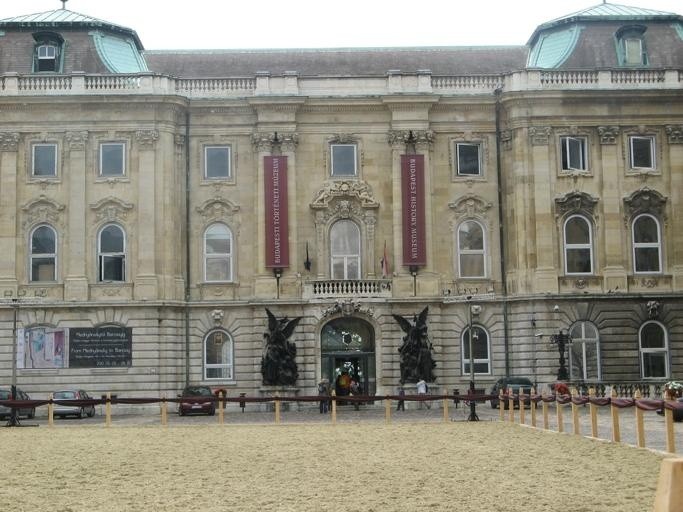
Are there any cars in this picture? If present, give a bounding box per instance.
[655,395,682,422]
[0,388,36,422]
[47,388,95,419]
[488,378,540,407]
[176,385,216,415]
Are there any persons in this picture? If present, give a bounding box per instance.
[351,380,360,411]
[395,379,405,412]
[416,376,431,410]
[317,379,330,414]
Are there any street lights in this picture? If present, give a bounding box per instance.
[8,298,19,426]
[548,325,577,397]
[465,298,480,422]
